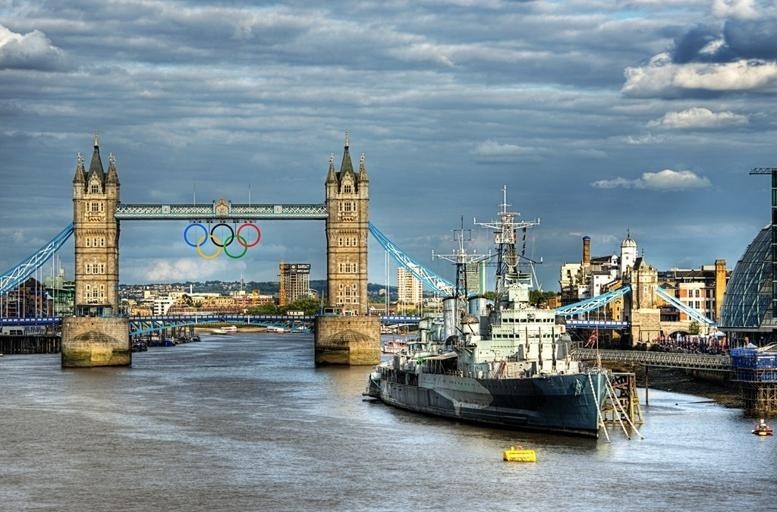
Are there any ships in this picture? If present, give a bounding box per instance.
[360,182,611,439]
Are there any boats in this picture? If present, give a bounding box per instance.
[502,444,536,464]
[210,326,239,336]
[751,417,772,437]
[131,335,200,352]
[266,326,308,334]
[381,339,406,355]
